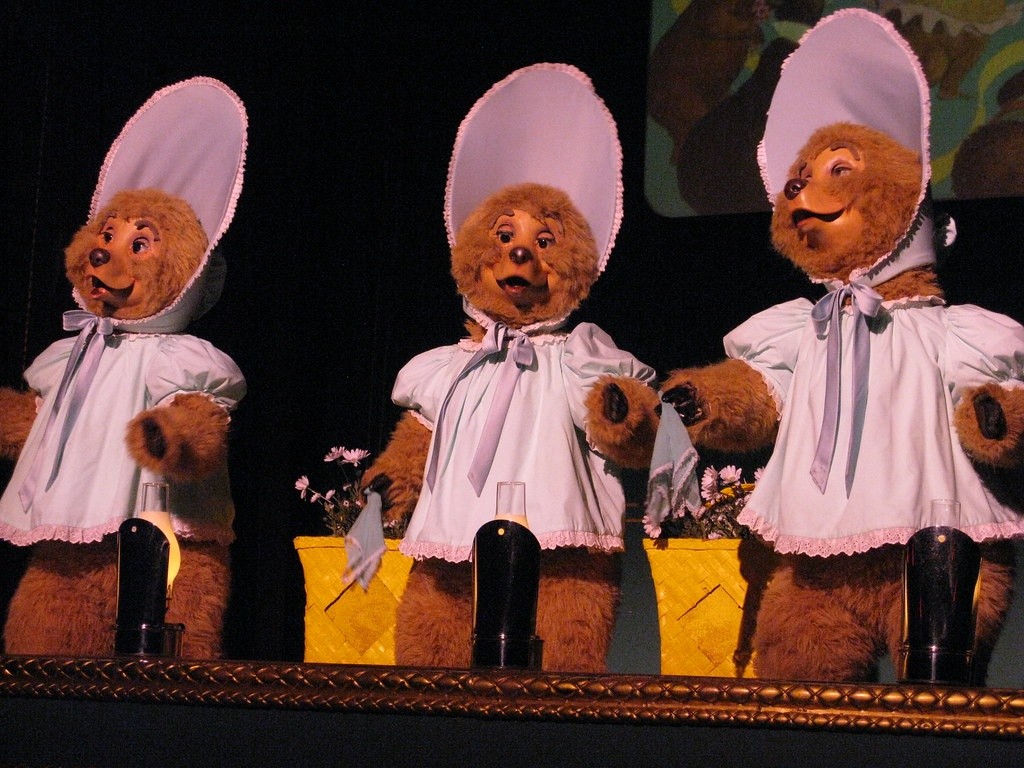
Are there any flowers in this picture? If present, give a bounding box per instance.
[293,442,408,537]
[646,464,782,538]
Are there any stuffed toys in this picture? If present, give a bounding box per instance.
[1,74,248,658]
[660,8,1023,691]
[357,62,660,671]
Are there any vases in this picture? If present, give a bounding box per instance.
[292,536,622,674]
[643,536,913,687]
